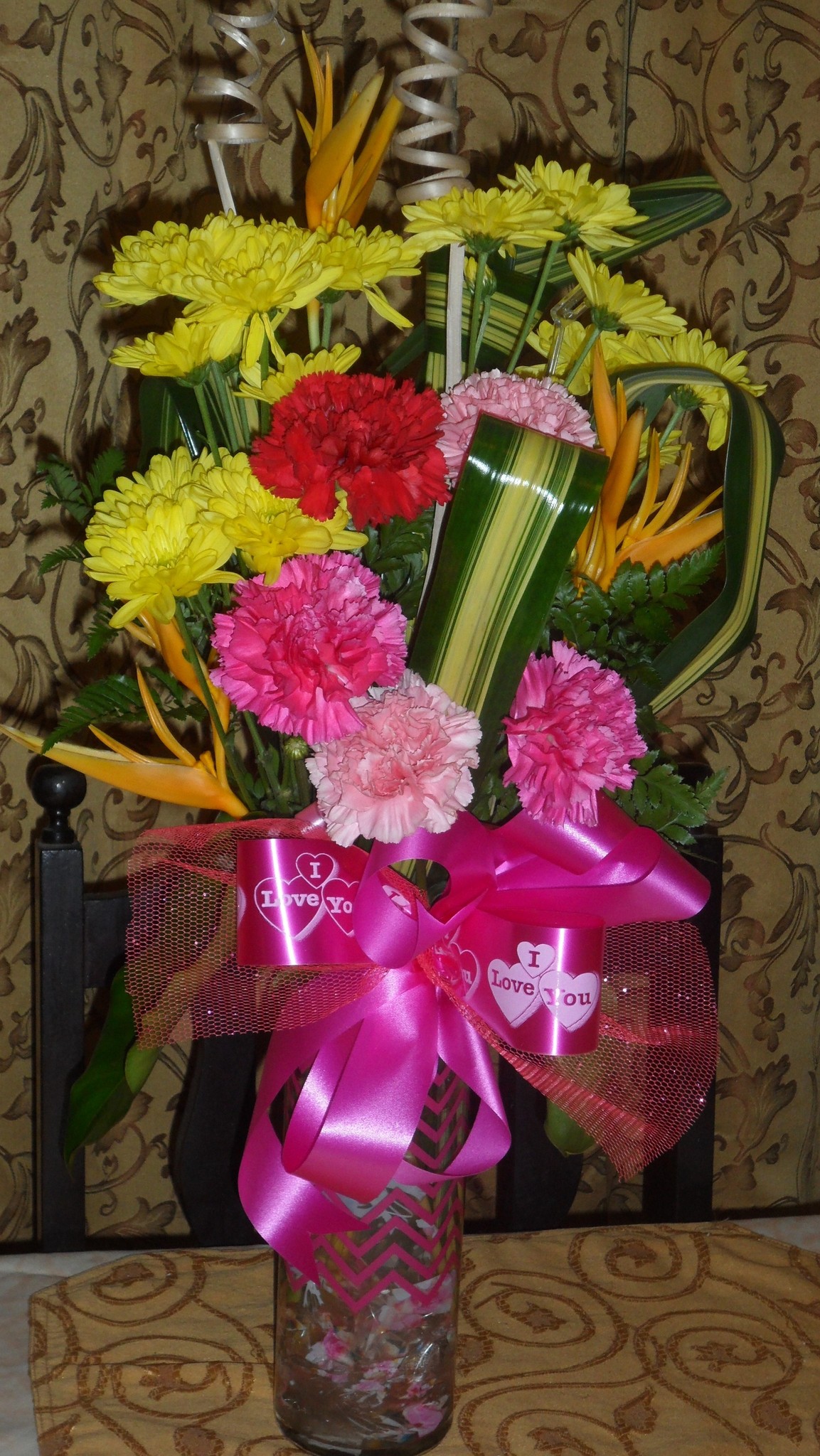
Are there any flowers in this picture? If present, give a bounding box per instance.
[2,0,789,1156]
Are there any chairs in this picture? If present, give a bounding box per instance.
[29,758,723,1259]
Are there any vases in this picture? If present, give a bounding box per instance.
[275,1018,470,1454]
